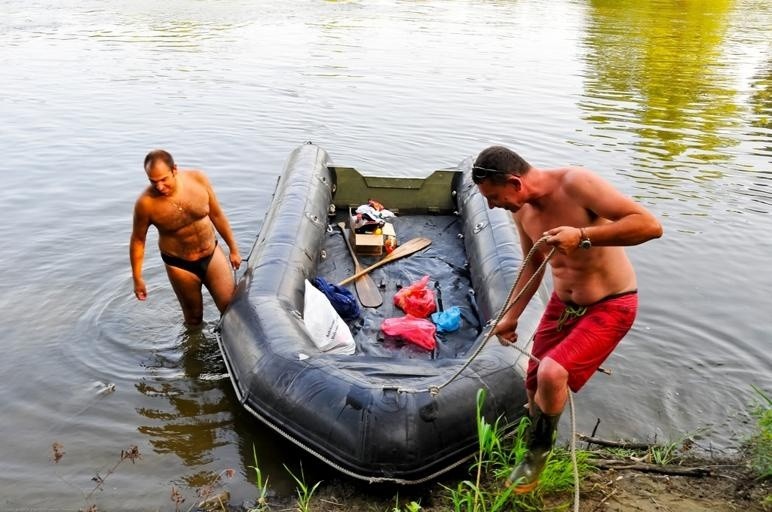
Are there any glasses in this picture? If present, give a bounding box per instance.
[471,166,521,179]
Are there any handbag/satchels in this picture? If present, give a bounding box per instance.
[313,277,360,321]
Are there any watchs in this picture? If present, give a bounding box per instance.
[579,227,592,250]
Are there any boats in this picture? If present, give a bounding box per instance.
[211,133,555,484]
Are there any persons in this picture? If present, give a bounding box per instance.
[471,147,664,491]
[129,150,241,326]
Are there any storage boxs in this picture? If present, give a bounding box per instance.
[347,207,384,256]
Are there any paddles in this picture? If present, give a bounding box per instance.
[337,221,383,308]
[336,237,431,287]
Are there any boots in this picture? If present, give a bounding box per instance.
[506,398,563,492]
[520,414,535,454]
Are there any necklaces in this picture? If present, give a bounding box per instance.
[161,174,184,212]
